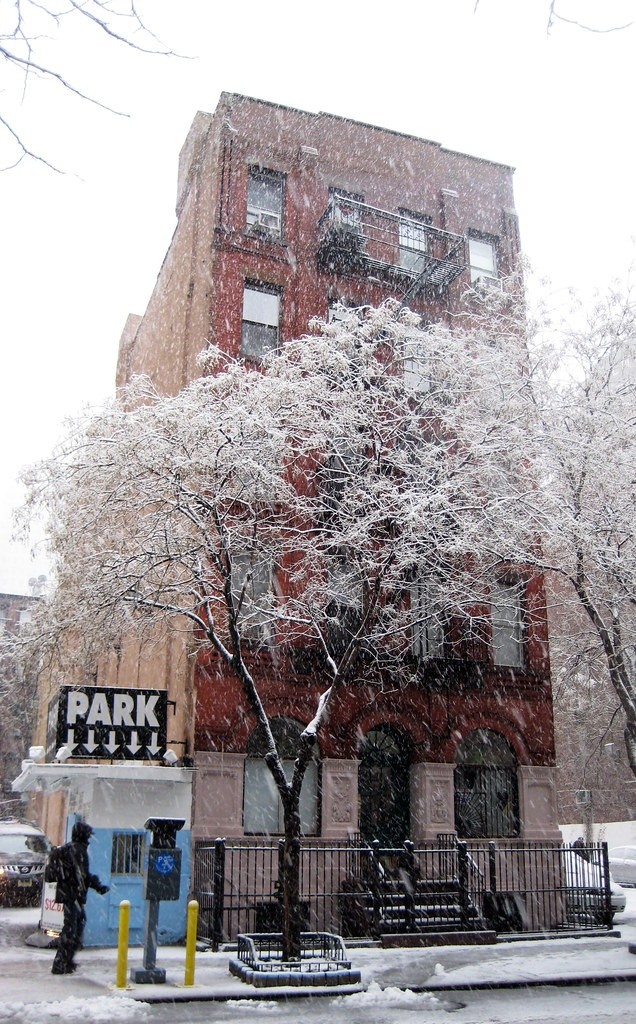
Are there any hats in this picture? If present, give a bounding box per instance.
[72,821,95,849]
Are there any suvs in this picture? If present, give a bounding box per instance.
[0,821,51,906]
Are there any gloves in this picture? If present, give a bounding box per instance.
[97,884,110,895]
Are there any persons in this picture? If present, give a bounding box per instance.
[51,821,110,975]
[572,836,588,863]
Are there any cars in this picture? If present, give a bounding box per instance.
[560,849,626,924]
[601,845,636,888]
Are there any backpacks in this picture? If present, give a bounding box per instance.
[44,844,74,883]
